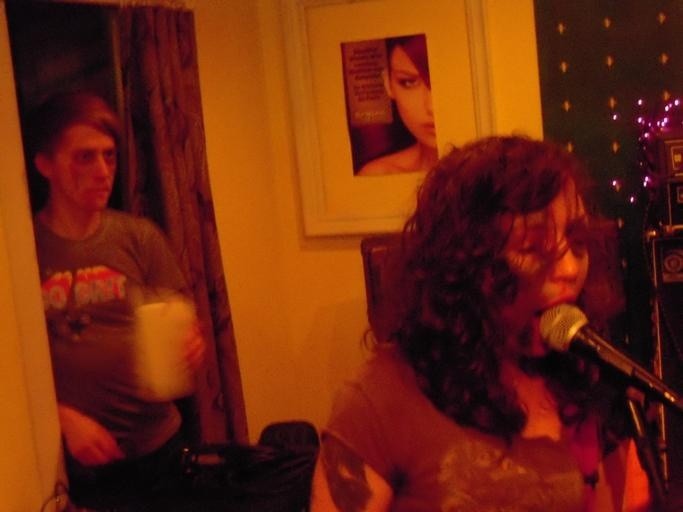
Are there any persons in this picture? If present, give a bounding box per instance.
[308,132,654,512]
[28,90,213,510]
[353,36,436,173]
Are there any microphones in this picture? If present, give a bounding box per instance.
[541,304,682,409]
